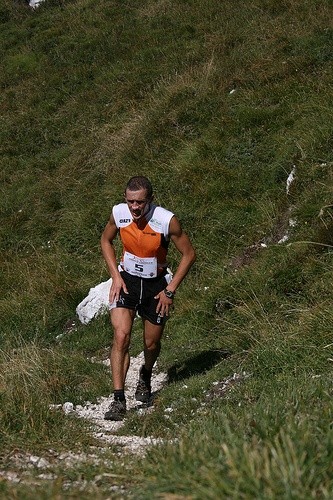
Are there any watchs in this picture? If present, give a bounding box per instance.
[164,288,174,299]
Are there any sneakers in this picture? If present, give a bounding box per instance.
[104,397,126,421]
[134,368,151,402]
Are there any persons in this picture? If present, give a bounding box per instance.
[100,175,195,421]
